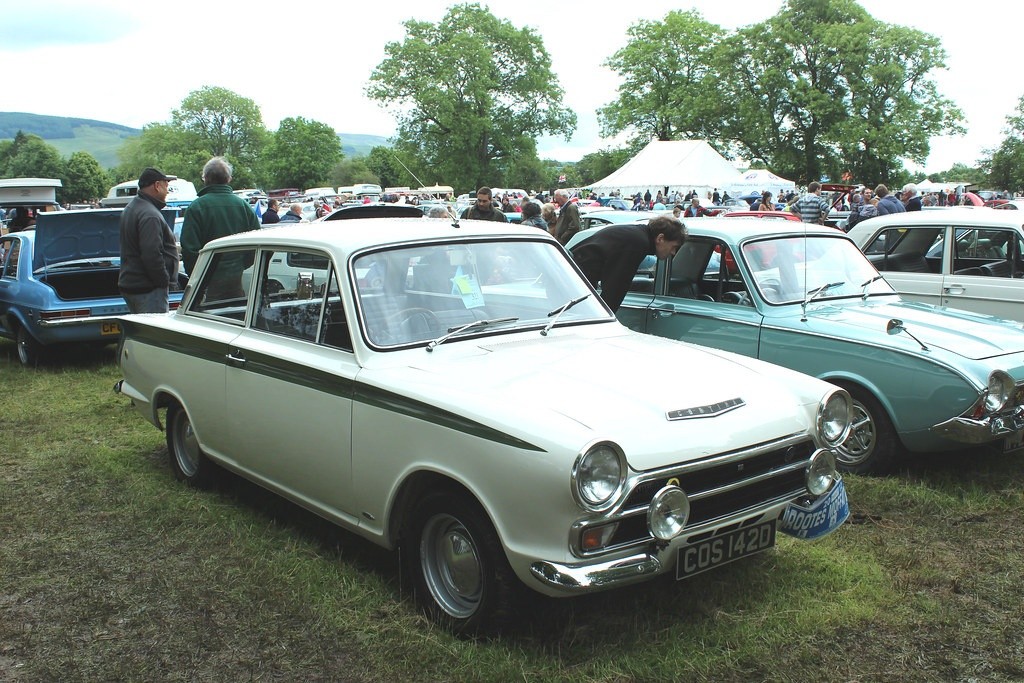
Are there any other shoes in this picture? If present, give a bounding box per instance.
[112,380,123,395]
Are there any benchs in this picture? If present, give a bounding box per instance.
[872,250,932,273]
[627,276,702,300]
[214,293,409,325]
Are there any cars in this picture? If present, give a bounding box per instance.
[707,191,1024,322]
[574,196,852,233]
[0,208,189,365]
[112,215,854,636]
[233,183,530,222]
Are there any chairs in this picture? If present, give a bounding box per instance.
[296,322,384,345]
[723,287,779,306]
[954,258,1013,275]
[401,309,490,336]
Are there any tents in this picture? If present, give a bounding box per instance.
[584,140,795,203]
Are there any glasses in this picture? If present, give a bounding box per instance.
[201,175,205,181]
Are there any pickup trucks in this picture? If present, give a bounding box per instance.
[475,216,1024,477]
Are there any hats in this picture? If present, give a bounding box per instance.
[138,168,176,188]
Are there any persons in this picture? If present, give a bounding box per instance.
[83,198,99,209]
[364,196,371,204]
[261,199,303,224]
[414,205,457,293]
[404,196,418,205]
[600,188,730,209]
[553,189,582,246]
[541,202,558,236]
[179,156,261,303]
[314,201,326,218]
[0,208,32,234]
[989,189,1010,200]
[495,190,550,213]
[444,194,450,201]
[460,187,509,285]
[750,188,798,212]
[673,198,723,216]
[790,182,830,226]
[118,168,179,314]
[568,216,689,314]
[833,183,960,231]
[520,202,548,231]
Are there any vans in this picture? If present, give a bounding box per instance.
[101,175,199,259]
